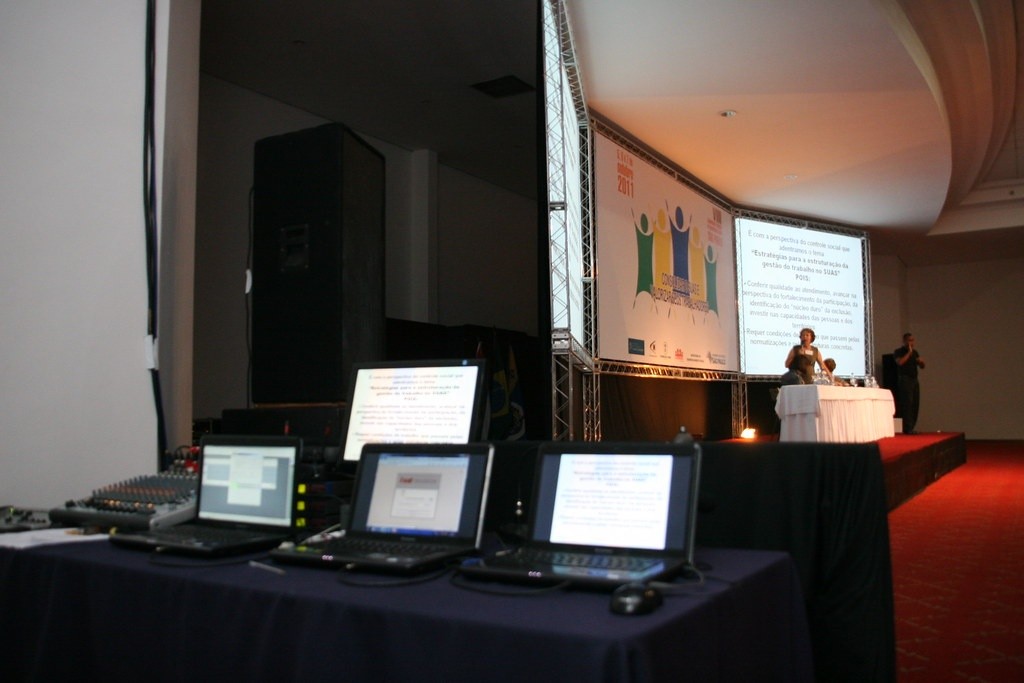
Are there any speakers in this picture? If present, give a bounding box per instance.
[251,122,384,406]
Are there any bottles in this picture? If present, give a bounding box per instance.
[870,377,876,387]
[850,373,855,385]
[822,369,829,385]
[864,377,869,387]
[816,369,821,384]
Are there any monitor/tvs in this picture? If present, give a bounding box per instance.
[340,357,484,465]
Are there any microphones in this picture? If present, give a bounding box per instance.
[801,341,806,349]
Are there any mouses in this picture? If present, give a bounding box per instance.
[611,582,665,616]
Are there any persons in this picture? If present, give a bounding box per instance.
[781,355,808,386]
[817,359,849,386]
[785,328,832,384]
[892,332,925,434]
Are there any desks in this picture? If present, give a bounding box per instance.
[774,384,896,443]
[0,509,816,683]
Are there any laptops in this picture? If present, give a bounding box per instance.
[456,442,701,588]
[109,436,303,561]
[269,443,495,576]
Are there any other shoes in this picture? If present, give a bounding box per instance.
[903,429,917,435]
